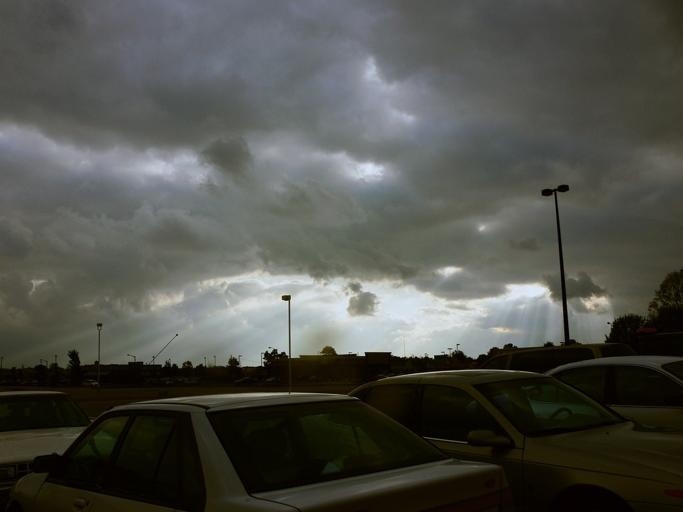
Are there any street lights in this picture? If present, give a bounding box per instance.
[96,322,102,378]
[540,184,569,346]
[281,294,291,393]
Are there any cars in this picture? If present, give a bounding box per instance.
[296,367,682,510]
[1,388,119,508]
[13,391,515,510]
[465,352,682,429]
